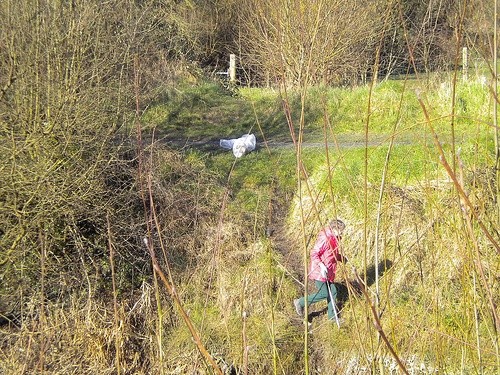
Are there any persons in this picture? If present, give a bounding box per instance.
[292,219,346,324]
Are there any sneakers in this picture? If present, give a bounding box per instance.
[329,318,345,324]
[294,299,303,316]
